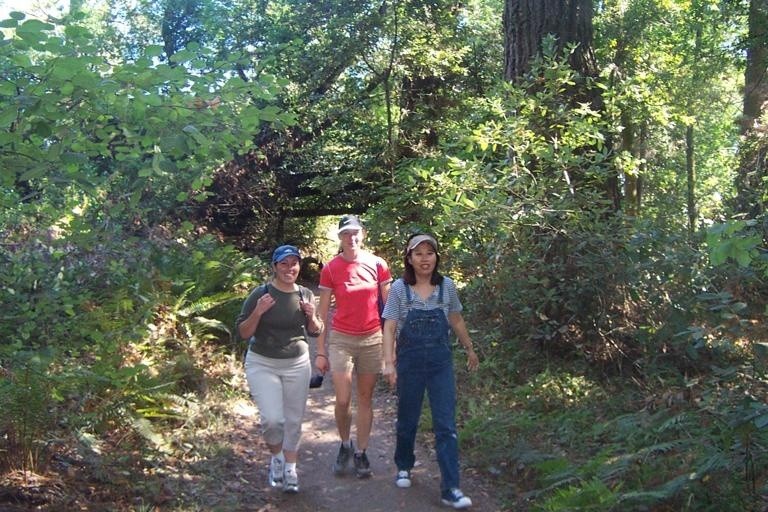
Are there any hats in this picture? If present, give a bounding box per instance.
[406,234,438,256]
[337,217,363,233]
[272,245,301,263]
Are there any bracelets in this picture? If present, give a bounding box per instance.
[464,343,473,350]
[316,354,328,359]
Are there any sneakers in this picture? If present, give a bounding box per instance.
[283,465,299,493]
[354,449,374,478]
[332,440,354,475]
[396,468,412,488]
[440,487,473,509]
[269,455,285,488]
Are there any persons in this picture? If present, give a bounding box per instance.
[380,233,480,509]
[234,245,325,494]
[316,215,393,480]
